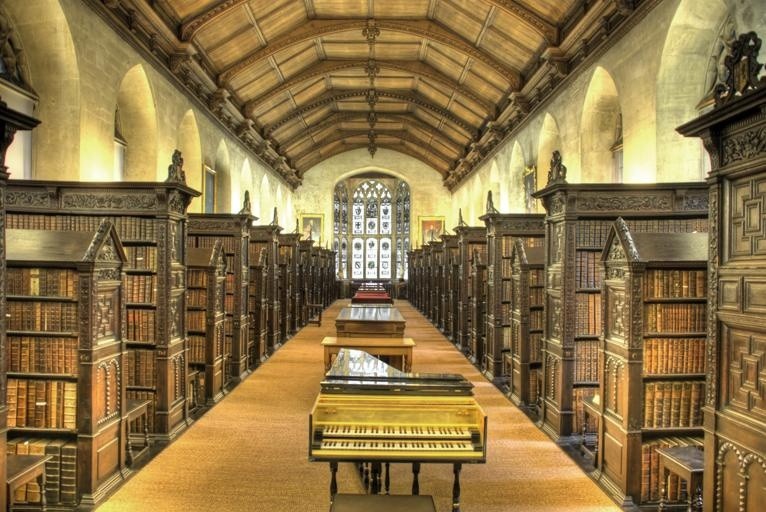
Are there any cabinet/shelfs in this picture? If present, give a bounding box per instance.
[184,213,337,422]
[599,261,709,512]
[530,183,709,470]
[1,177,204,469]
[674,88,766,512]
[1,260,126,512]
[408,214,545,420]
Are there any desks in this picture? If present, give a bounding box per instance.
[320,283,415,372]
[306,374,486,512]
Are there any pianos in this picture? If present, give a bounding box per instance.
[310,348,486,512]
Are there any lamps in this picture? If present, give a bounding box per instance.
[363,1,380,159]
